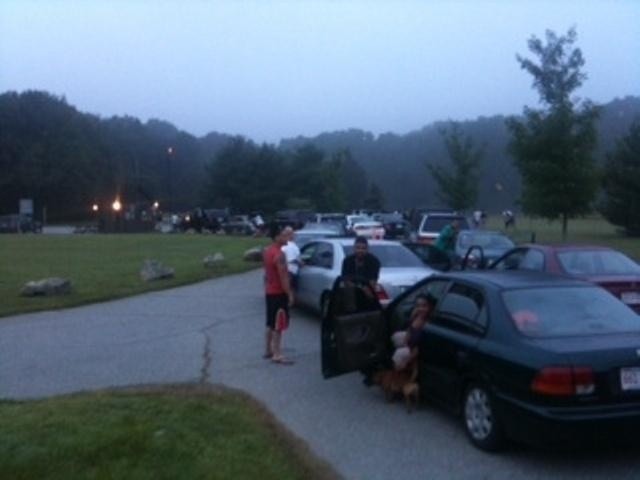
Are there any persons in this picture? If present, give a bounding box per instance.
[156,207,222,235]
[432,219,461,252]
[453,207,469,228]
[388,292,438,383]
[261,224,299,365]
[472,208,485,226]
[502,209,516,229]
[395,208,425,226]
[280,231,304,307]
[339,235,381,300]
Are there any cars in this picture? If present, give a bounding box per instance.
[289,238,453,322]
[0,213,44,234]
[487,243,640,317]
[318,271,640,449]
[181,206,471,262]
[449,228,518,271]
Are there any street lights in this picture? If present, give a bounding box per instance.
[165,147,176,211]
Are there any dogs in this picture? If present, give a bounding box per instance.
[362,361,419,415]
